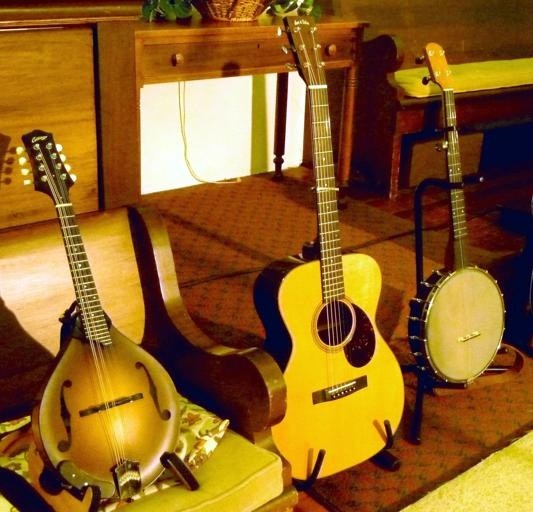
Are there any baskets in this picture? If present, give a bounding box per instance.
[190,0,277,21]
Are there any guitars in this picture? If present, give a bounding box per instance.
[252,15,405,479]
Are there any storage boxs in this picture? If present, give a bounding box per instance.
[402,132,481,188]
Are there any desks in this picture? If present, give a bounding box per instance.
[134,13,362,209]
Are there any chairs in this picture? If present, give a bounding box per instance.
[0,201,297,512]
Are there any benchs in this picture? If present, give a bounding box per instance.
[327,31,532,201]
